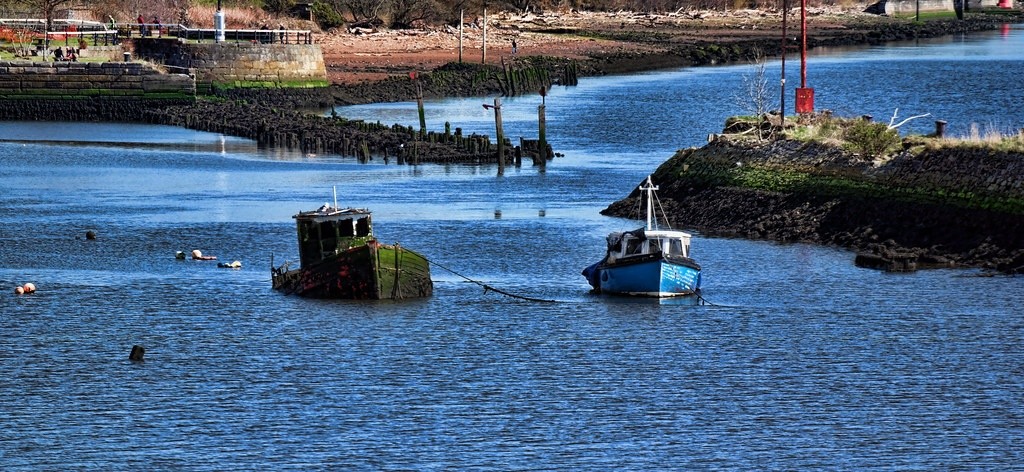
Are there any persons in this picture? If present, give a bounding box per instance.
[511,39,517,54]
[191,249,217,260]
[217,260,242,269]
[152,16,161,29]
[54,46,78,62]
[137,13,146,38]
[260,24,270,30]
[279,23,285,44]
[108,15,115,30]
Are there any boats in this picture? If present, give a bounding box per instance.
[582,174,701,298]
[272,186,433,300]
